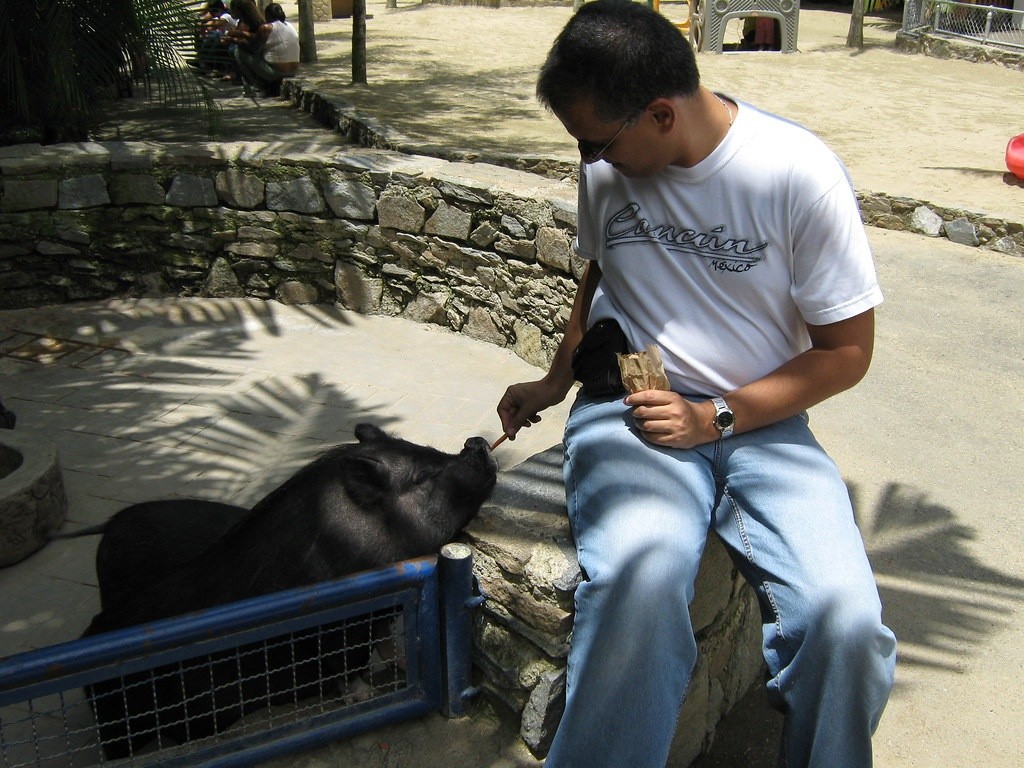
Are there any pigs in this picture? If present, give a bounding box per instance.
[39,422,498,762]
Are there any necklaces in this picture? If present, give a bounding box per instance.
[712,93,733,128]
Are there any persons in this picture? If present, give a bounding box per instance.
[497,0,895,768]
[739,16,775,51]
[185,0,300,98]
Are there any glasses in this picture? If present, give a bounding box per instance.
[578,120,630,164]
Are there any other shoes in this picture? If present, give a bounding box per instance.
[220,72,236,82]
[242,89,265,97]
[186,60,200,66]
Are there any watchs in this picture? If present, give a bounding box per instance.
[708,398,736,440]
